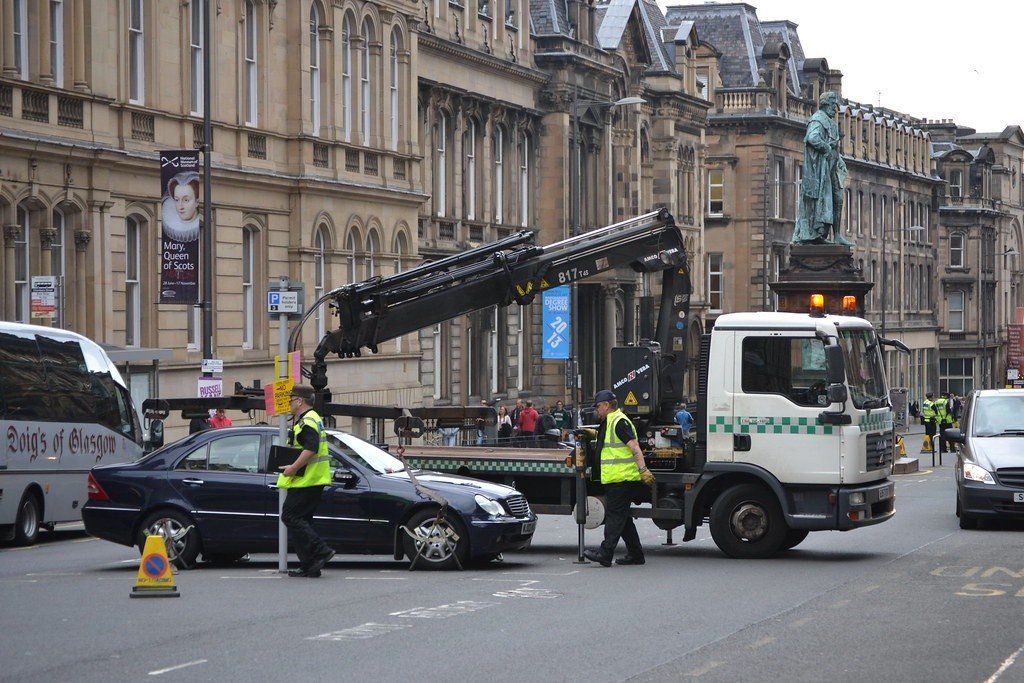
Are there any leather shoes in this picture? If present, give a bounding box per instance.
[307,547,336,575]
[583,549,612,567]
[287,565,322,578]
[615,553,645,565]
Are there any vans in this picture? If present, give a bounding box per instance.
[0,321,163,548]
[944,388,1024,528]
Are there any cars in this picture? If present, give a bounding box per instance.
[80,424,539,572]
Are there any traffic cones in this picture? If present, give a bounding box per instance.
[920,434,936,453]
[129,536,180,597]
[897,437,907,457]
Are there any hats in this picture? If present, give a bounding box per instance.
[591,390,617,407]
[926,392,934,399]
[942,392,949,397]
[284,384,316,399]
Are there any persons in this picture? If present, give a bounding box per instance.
[912,392,965,452]
[439,428,459,446]
[476,399,586,446]
[674,403,694,435]
[211,408,232,428]
[277,385,335,577]
[793,91,854,246]
[583,390,655,567]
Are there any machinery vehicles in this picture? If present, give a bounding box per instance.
[281,205,705,470]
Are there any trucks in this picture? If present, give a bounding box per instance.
[330,294,911,559]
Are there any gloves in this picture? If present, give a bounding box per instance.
[639,466,657,487]
[573,428,598,441]
